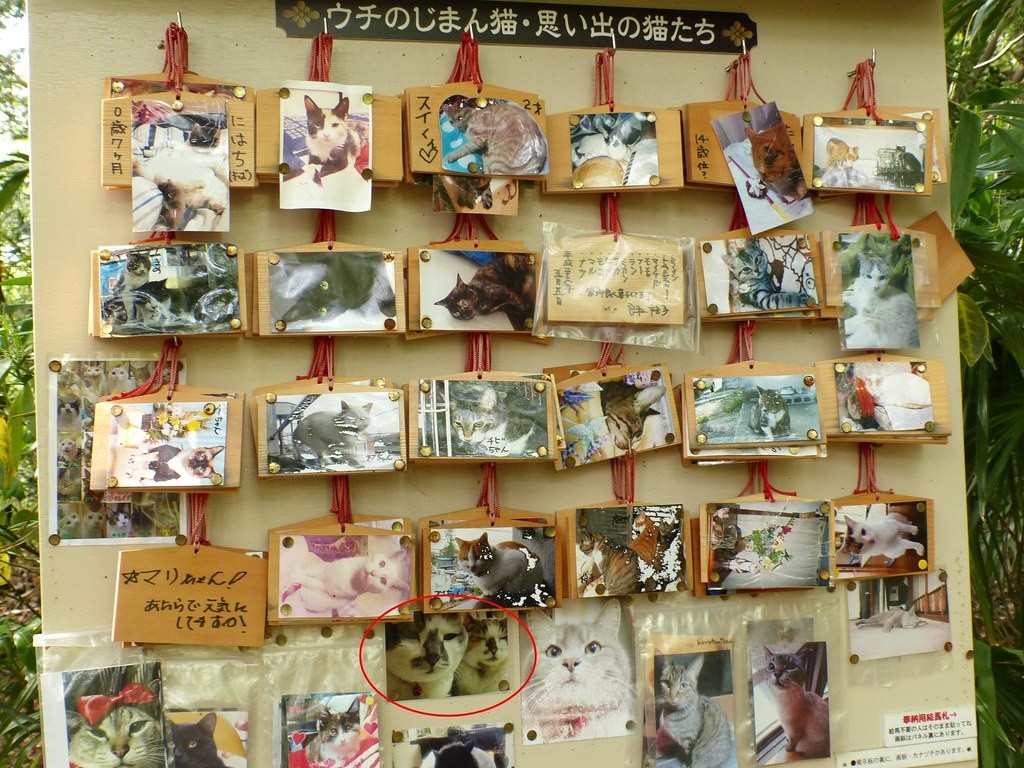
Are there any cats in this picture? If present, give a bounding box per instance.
[43,76,940,768]
